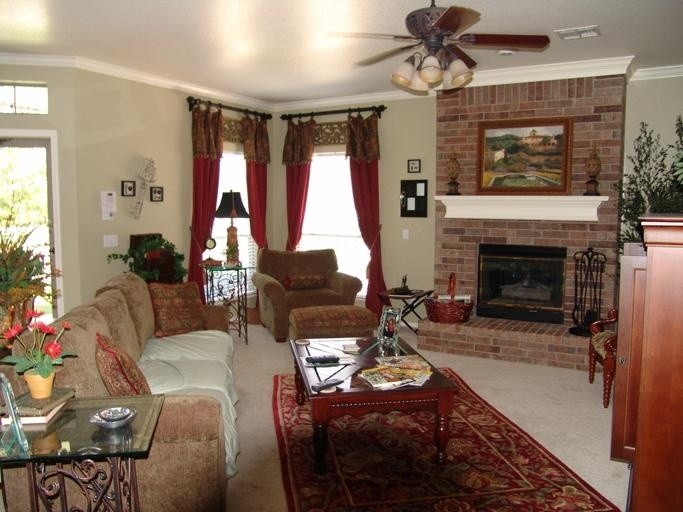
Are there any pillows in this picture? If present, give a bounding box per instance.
[150,282,206,337]
[96,333,152,397]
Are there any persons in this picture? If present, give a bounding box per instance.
[383,318,395,338]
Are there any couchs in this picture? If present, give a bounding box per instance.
[0,271,234,511]
[253,249,362,342]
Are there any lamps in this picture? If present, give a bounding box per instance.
[215,190,250,267]
[390,44,473,96]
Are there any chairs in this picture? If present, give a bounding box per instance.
[587,309,616,408]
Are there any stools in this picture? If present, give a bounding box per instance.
[288,305,377,340]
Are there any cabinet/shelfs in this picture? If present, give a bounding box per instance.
[610,214,683,512]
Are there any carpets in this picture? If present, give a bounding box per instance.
[272,369,620,512]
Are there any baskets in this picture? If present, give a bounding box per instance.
[424,272,473,323]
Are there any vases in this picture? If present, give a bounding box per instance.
[24,368,55,399]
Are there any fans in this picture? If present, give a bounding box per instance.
[327,1,549,70]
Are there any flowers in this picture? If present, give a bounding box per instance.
[2,309,78,378]
[1,150,65,326]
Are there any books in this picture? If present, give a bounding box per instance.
[1,400,68,426]
[4,388,75,416]
[357,355,434,391]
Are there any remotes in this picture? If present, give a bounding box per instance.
[311,378,345,393]
[306,354,340,363]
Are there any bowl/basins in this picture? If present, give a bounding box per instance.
[98,405,131,422]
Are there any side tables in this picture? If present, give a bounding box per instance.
[381,290,432,335]
[204,264,254,345]
[0,395,166,511]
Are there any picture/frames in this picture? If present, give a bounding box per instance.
[408,159,420,173]
[121,181,135,196]
[476,118,573,196]
[151,187,164,201]
[1,375,30,454]
[377,305,403,348]
